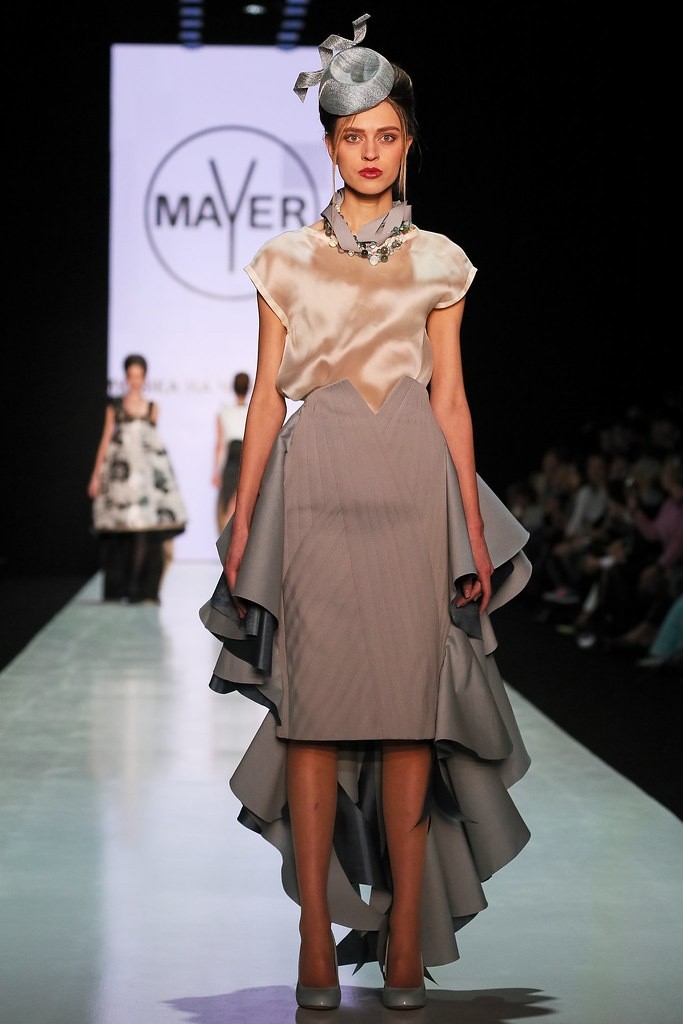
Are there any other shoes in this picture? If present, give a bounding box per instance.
[559,623,584,637]
[641,657,667,668]
[545,592,580,605]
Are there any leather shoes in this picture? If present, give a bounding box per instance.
[383,934,427,1008]
[296,930,341,1009]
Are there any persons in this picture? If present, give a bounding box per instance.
[86,354,187,606]
[503,408,682,671]
[209,13,494,1010]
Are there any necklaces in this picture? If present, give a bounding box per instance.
[321,186,412,266]
